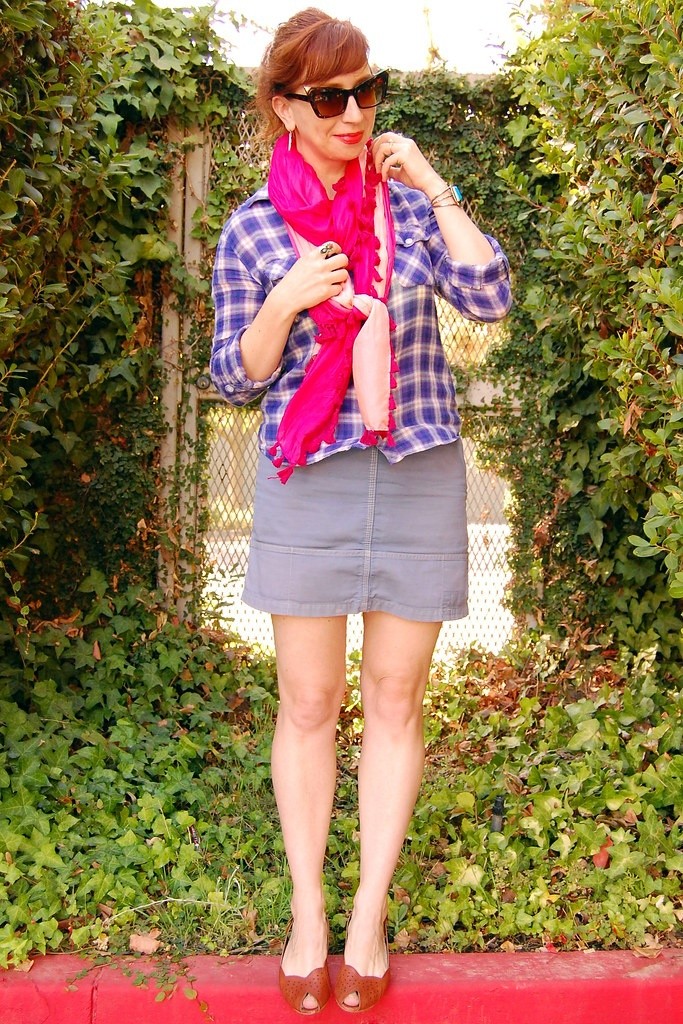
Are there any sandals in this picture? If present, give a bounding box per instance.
[279,912,332,1015]
[335,914,390,1012]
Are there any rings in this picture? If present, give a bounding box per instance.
[397,132,404,143]
[319,242,334,257]
[388,138,395,153]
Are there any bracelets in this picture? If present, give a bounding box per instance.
[430,184,464,209]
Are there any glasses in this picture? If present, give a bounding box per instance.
[283,68,389,119]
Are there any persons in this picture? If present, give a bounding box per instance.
[208,5,516,1016]
